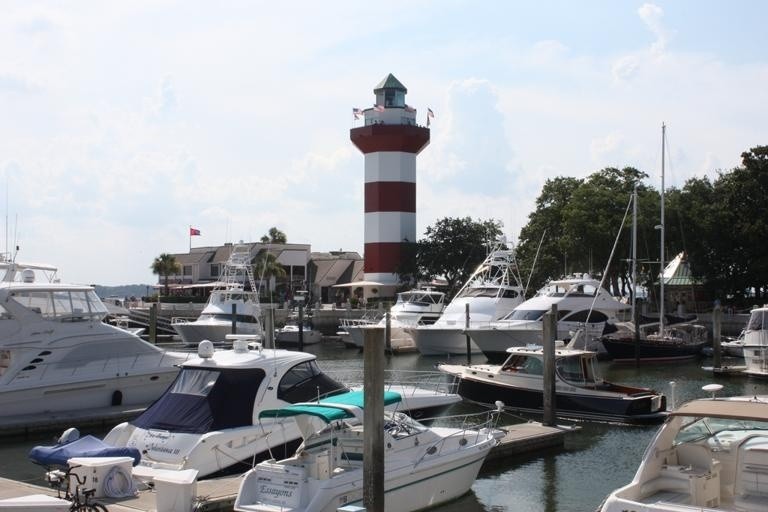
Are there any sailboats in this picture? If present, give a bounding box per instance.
[598,121,710,365]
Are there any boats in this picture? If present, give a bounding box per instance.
[403,234,526,357]
[28,333,462,488]
[171,240,279,349]
[459,270,640,363]
[597,383,768,511]
[742,304,767,378]
[104,313,146,339]
[335,282,448,353]
[435,328,673,427]
[233,409,508,511]
[1,240,226,440]
[720,330,745,358]
[273,323,325,346]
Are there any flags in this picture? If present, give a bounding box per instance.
[190,228,200,236]
